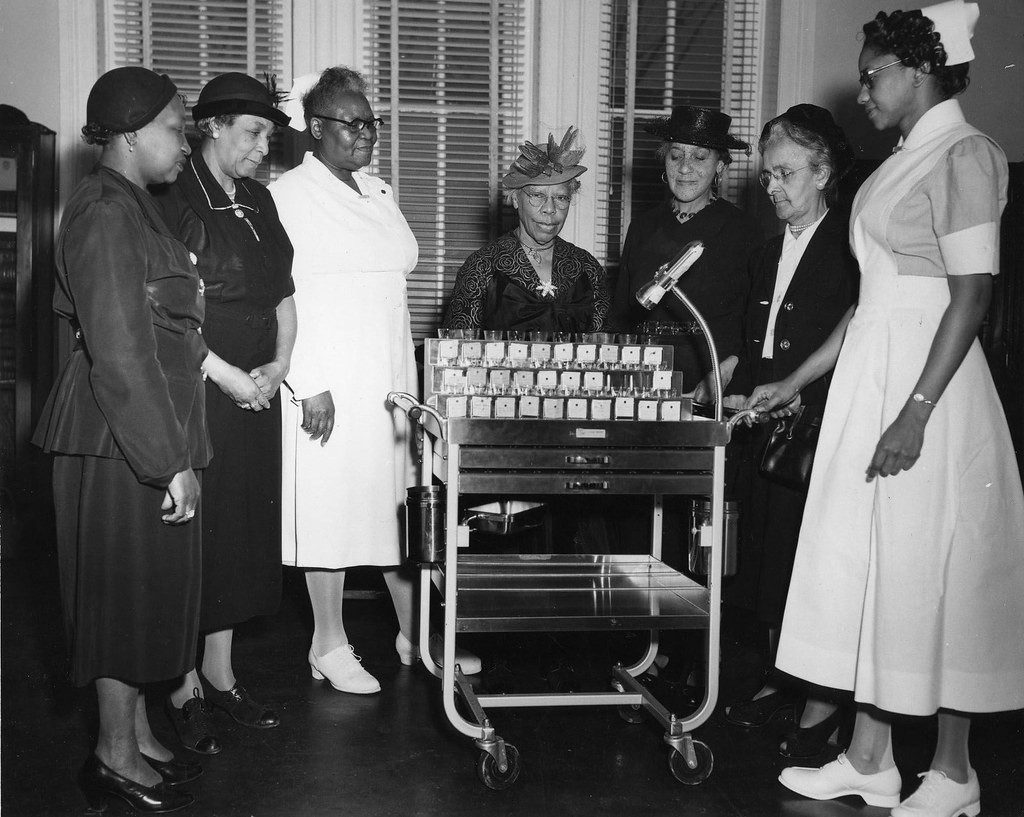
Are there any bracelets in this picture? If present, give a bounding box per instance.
[909,393,936,407]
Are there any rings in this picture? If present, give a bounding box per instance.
[186,510,195,518]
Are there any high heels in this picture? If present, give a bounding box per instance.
[81,754,196,814]
[724,684,805,726]
[309,645,381,694]
[199,671,281,728]
[396,630,482,675]
[140,749,204,785]
[167,691,222,754]
[779,701,848,757]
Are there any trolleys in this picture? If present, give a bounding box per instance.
[388,391,729,794]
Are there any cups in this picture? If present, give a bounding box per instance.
[484,330,503,340]
[641,335,660,344]
[462,329,480,339]
[407,486,447,562]
[438,328,456,339]
[507,331,525,340]
[618,334,638,344]
[553,332,571,342]
[575,332,594,343]
[688,498,739,577]
[597,334,615,343]
[529,331,548,341]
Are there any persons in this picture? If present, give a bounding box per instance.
[683,102,867,762]
[31,68,212,813]
[443,126,613,341]
[142,73,297,755]
[261,66,484,697]
[610,102,753,710]
[771,0,1024,817]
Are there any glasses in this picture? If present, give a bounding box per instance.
[521,188,573,209]
[759,164,817,187]
[860,57,909,88]
[314,115,384,132]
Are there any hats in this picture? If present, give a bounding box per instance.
[87,67,177,131]
[502,126,587,188]
[762,104,850,171]
[645,106,749,150]
[920,0,979,66]
[193,72,291,127]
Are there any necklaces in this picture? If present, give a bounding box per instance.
[226,183,236,196]
[671,191,719,220]
[789,222,814,232]
[518,240,553,265]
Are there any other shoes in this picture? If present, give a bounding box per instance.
[891,770,981,817]
[778,751,902,807]
[683,658,706,707]
[640,653,671,685]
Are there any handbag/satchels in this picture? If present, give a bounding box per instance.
[761,408,822,489]
